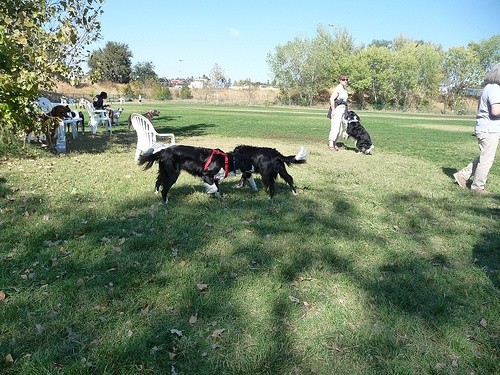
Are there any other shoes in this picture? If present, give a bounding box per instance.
[329,145,339,151]
[453,171,467,189]
[471,187,493,195]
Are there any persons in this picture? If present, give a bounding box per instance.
[452,61,500,195]
[327,73,350,153]
[137,93,142,106]
[92,91,118,126]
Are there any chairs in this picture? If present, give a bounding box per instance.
[130,113,176,162]
[26,96,113,151]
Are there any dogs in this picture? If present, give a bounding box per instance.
[127,110,161,132]
[138,144,309,204]
[343,111,375,155]
[110,108,125,127]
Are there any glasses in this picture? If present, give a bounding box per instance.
[340,78,349,81]
